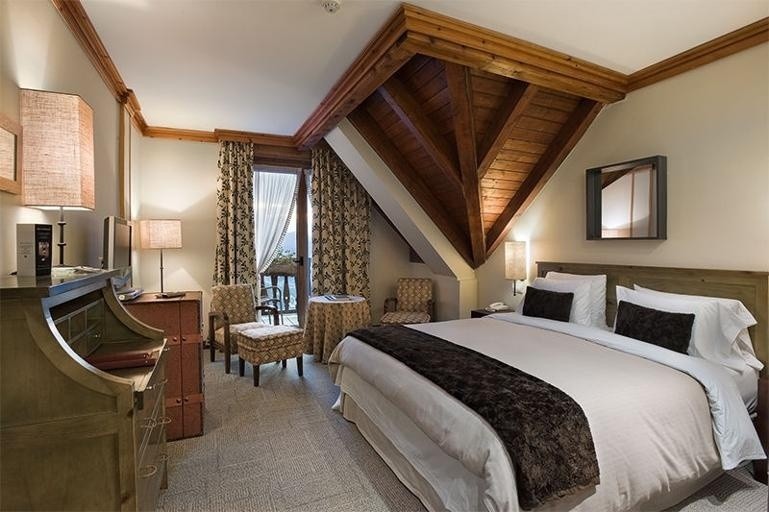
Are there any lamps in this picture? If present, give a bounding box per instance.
[140,219,183,293]
[505,241,527,295]
[17,85,97,271]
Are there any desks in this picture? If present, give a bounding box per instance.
[302,292,369,364]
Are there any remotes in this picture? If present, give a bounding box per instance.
[162,291,185,298]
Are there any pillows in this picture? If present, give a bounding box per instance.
[533,278,596,326]
[616,299,694,355]
[523,284,573,324]
[544,269,607,329]
[613,283,741,372]
[633,283,764,373]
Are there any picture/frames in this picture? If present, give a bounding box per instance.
[0,114,23,197]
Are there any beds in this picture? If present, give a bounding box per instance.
[325,256,769,511]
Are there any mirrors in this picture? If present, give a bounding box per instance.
[585,151,668,239]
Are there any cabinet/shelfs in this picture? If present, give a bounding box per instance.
[1,263,172,511]
[121,287,207,442]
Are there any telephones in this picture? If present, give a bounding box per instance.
[488,302,509,310]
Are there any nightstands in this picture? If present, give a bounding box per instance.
[471,307,514,320]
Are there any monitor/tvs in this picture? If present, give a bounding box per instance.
[104,216,135,294]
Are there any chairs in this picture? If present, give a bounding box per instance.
[256,284,285,324]
[380,277,438,323]
[207,283,305,386]
